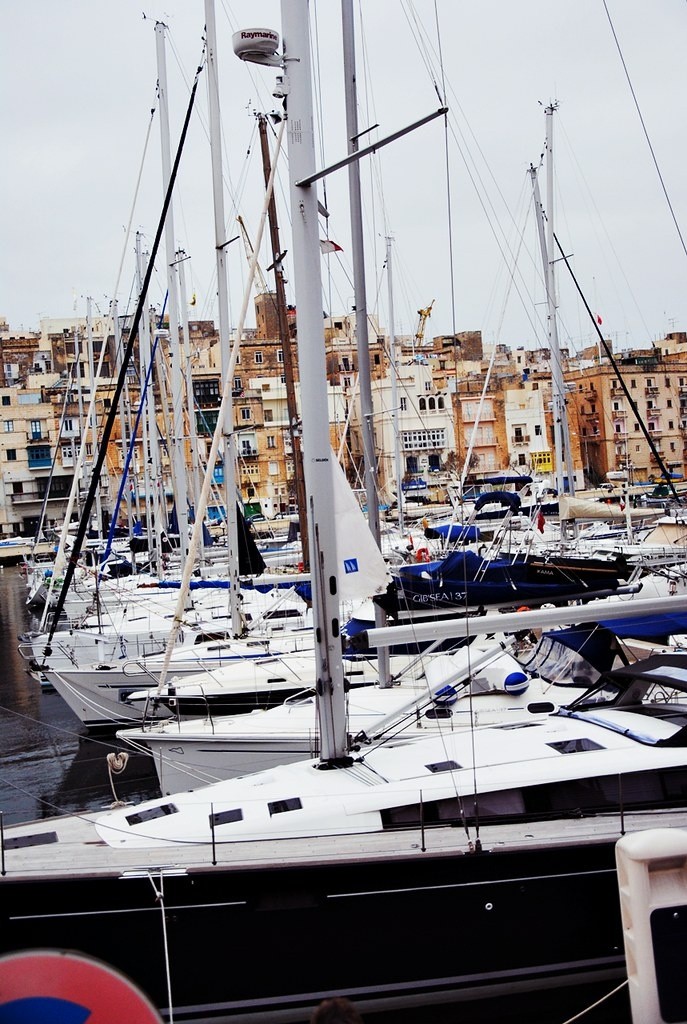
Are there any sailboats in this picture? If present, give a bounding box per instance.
[0,0,687,1020]
